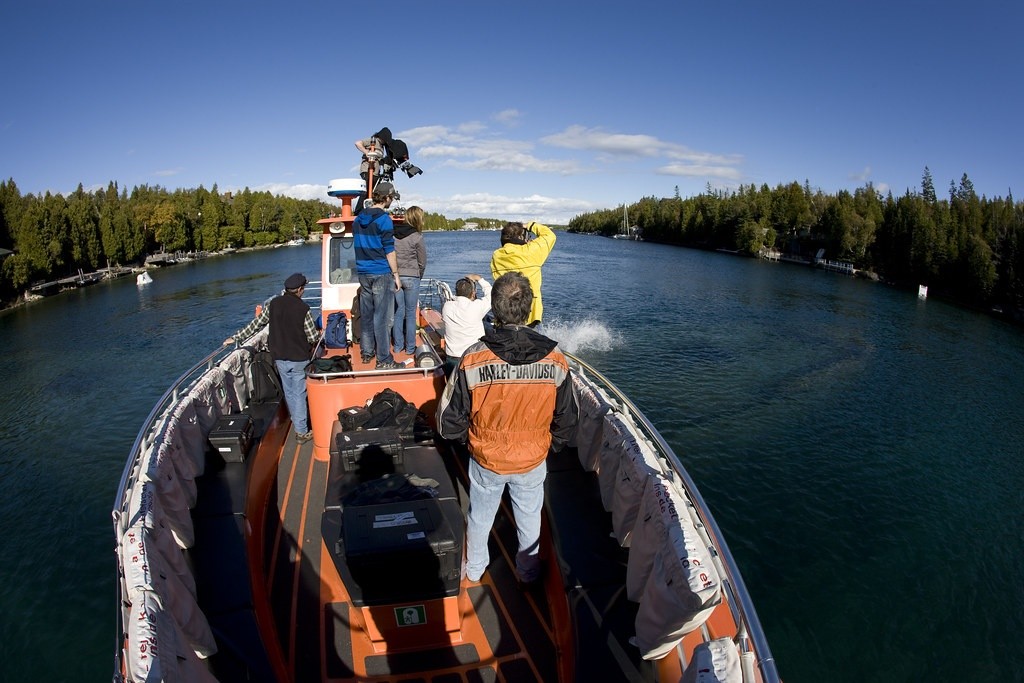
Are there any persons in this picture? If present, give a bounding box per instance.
[353,127,392,216]
[490,221,556,328]
[352,182,406,370]
[436,270,580,582]
[393,206,426,355]
[441,273,493,382]
[223,273,324,444]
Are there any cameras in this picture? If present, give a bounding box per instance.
[523,228,531,243]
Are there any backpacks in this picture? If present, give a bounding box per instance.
[241,346,283,401]
[362,387,427,433]
[325,312,353,353]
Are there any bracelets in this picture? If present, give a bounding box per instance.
[393,272,398,274]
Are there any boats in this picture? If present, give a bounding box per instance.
[136,272,153,285]
[111,135,782,683]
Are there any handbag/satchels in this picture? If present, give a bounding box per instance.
[305,355,354,376]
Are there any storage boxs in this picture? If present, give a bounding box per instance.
[335,426,404,472]
[320,419,460,607]
[337,405,373,431]
[208,414,256,464]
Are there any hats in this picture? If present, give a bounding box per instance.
[285,273,309,289]
[374,183,400,200]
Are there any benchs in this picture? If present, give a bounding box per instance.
[535,449,660,683]
[189,392,289,683]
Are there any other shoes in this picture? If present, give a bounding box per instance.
[295,430,313,445]
[376,359,405,371]
[361,351,375,363]
